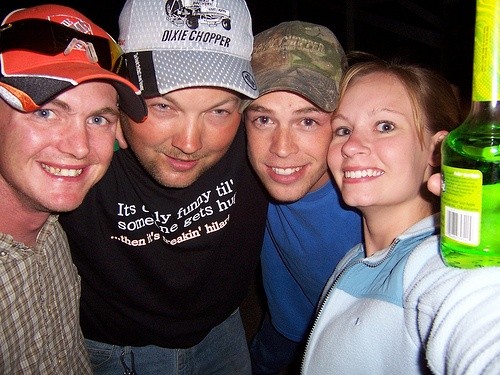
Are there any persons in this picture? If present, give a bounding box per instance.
[59,0,270,375]
[301,58,499,375]
[244,20,364,375]
[1,4,148,375]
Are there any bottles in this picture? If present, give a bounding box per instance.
[439,0,500,267]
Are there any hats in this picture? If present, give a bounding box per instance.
[239,21,347,112]
[0,5,147,124]
[118,0,259,99]
